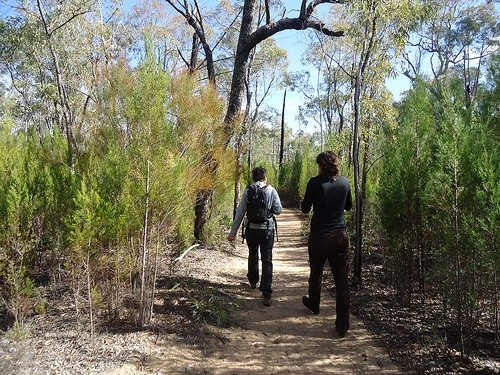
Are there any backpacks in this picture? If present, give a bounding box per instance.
[246,184,270,224]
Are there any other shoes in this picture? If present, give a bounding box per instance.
[251,283,256,288]
[262,294,273,306]
[338,326,346,337]
[302,296,319,314]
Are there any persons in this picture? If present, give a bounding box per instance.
[228,167,282,305]
[301,152,353,337]
